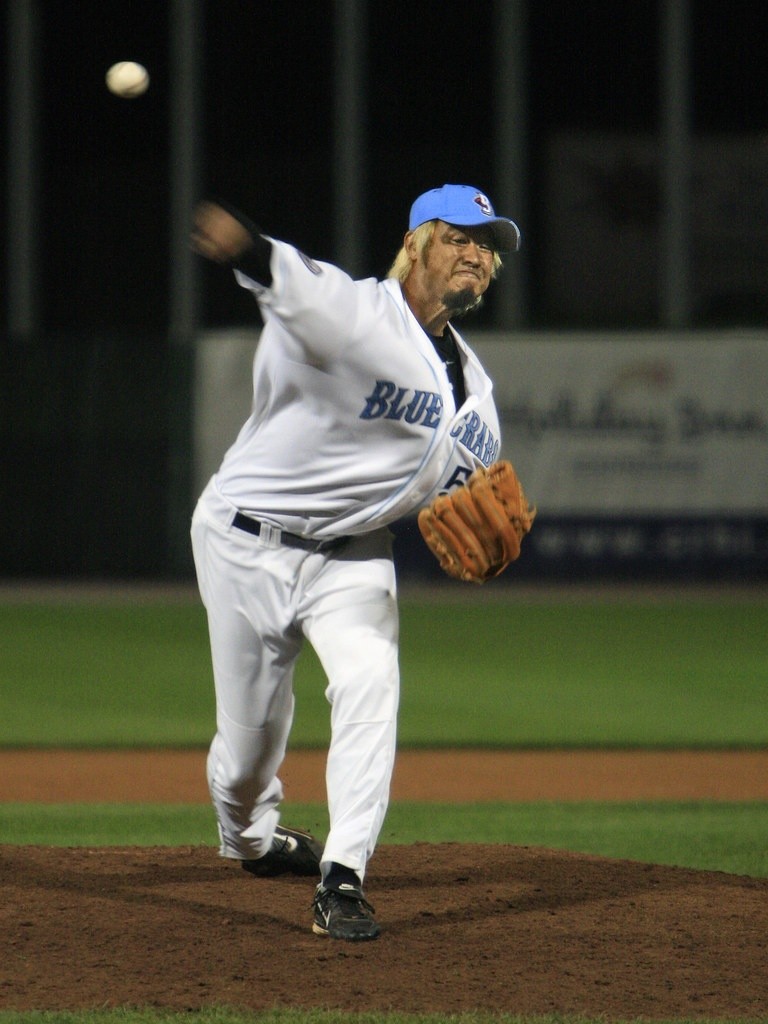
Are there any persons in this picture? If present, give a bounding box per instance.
[188,183,536,940]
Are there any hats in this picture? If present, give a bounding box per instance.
[410,183,520,255]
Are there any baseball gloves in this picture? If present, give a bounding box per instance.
[410,458,540,585]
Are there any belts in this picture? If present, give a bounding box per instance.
[232,511,347,554]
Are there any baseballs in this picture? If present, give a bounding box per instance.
[104,59,151,99]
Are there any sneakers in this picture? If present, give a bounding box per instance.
[242,824,314,877]
[309,878,380,940]
